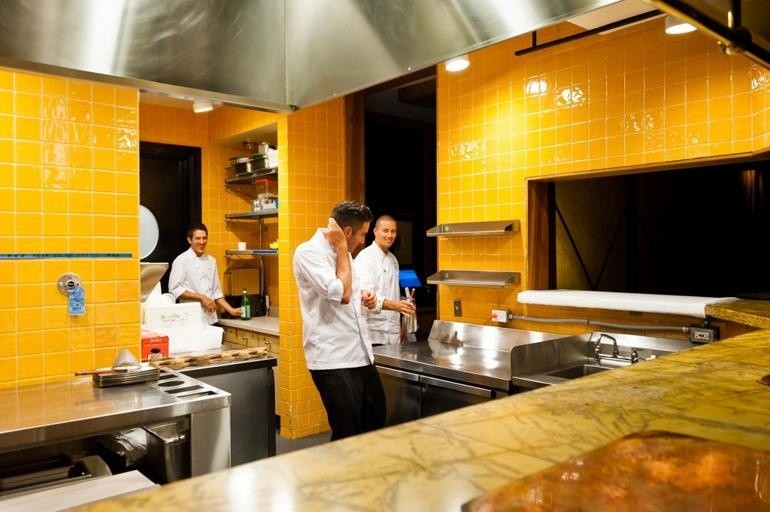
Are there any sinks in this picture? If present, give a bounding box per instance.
[547,365,612,380]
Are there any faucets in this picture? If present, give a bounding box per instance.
[594,333,618,359]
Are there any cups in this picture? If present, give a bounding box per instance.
[238,241,247,251]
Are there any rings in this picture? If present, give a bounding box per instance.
[404,305,407,309]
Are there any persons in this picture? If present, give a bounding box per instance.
[168,223,243,326]
[354,215,416,347]
[294,199,386,442]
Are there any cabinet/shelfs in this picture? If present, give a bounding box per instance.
[225,169,277,259]
[423,219,523,290]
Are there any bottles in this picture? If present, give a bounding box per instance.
[240,289,251,321]
[401,298,419,334]
